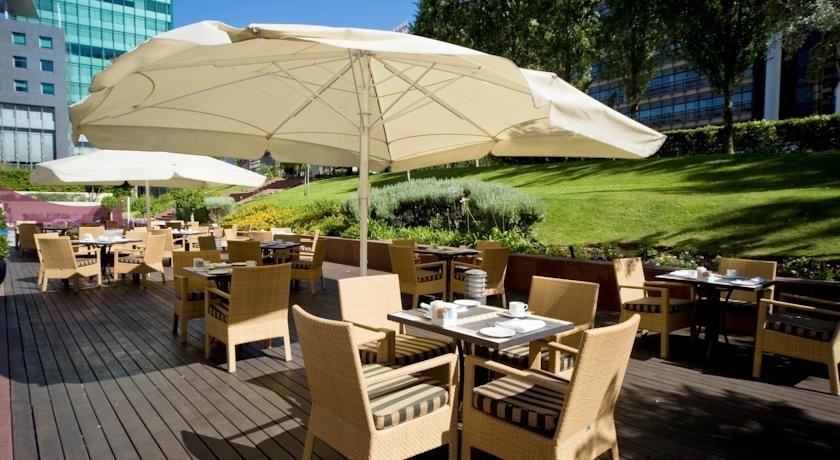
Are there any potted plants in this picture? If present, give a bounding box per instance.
[0,208,10,283]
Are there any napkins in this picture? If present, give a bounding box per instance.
[493,318,546,334]
[430,301,469,313]
[671,270,696,279]
[731,279,758,285]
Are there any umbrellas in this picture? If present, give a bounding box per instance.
[28,150,267,230]
[67,20,668,276]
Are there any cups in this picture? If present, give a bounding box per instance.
[439,246,443,251]
[509,301,528,315]
[460,245,465,251]
[703,271,712,278]
[443,305,457,327]
[287,242,294,246]
[276,239,282,244]
[204,261,209,270]
[697,267,706,277]
[268,241,273,245]
[247,261,257,267]
[727,269,737,278]
[431,301,445,324]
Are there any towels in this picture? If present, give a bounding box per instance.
[494,318,546,334]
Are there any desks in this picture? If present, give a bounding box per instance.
[71,239,143,284]
[42,227,70,236]
[655,274,774,361]
[182,265,234,304]
[259,241,302,263]
[170,229,207,250]
[388,304,575,409]
[410,247,479,300]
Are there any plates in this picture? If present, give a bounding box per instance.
[502,310,531,318]
[721,276,743,280]
[454,299,481,306]
[480,327,515,337]
[731,281,755,285]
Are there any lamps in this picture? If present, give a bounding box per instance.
[465,269,488,305]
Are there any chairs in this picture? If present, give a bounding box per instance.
[612,257,840,395]
[13,219,510,372]
[291,276,641,460]
